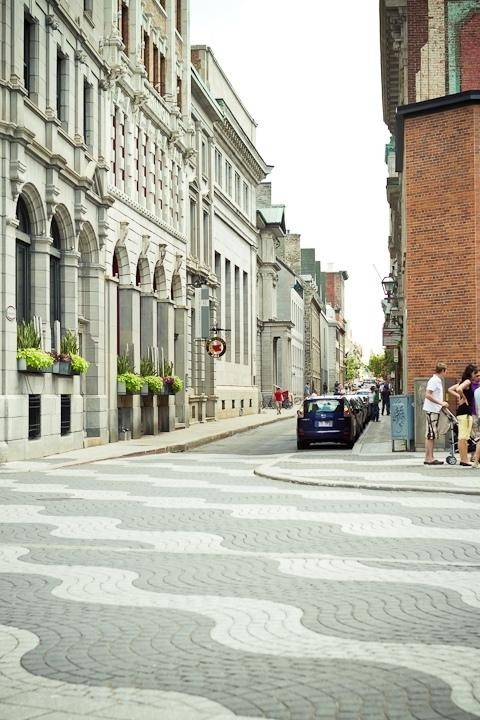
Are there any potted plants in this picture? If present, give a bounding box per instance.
[17,320,90,376]
[117,352,183,396]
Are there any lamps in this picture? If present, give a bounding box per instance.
[381,276,403,327]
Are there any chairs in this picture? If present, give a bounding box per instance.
[311,404,341,412]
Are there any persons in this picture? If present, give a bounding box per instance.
[469,368,480,463]
[447,363,478,466]
[376,382,380,391]
[370,390,380,423]
[368,386,376,421]
[381,384,390,416]
[304,383,310,399]
[422,363,450,465]
[471,387,480,469]
[334,380,339,393]
[312,389,318,396]
[275,388,284,414]
[323,382,328,396]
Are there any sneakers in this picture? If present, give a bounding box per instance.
[422,458,480,470]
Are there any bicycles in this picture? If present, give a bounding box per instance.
[260,396,302,409]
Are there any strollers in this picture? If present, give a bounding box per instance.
[442,405,480,465]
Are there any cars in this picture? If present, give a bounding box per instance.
[296,379,382,448]
